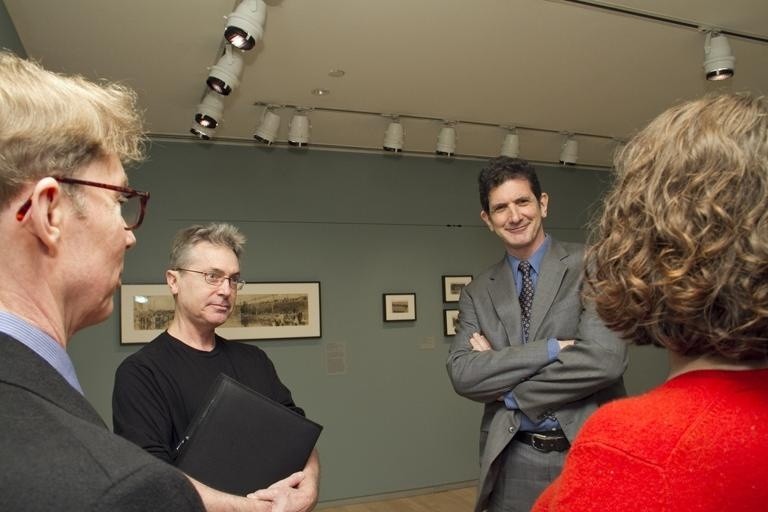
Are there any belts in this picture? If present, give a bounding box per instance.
[515,431,570,453]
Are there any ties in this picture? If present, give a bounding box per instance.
[517,259,536,341]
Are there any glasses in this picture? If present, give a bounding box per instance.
[16,176,150,230]
[171,268,246,290]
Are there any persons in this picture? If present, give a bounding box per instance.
[112,223,324,511]
[446,156,628,511]
[0,47,208,511]
[531,87,768,512]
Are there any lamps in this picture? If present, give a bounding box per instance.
[191,124,215,141]
[224,1,268,53]
[206,41,246,97]
[435,119,457,158]
[288,106,311,147]
[254,104,284,146]
[382,115,404,154]
[502,125,520,157]
[702,28,735,82]
[194,90,225,130]
[559,133,579,165]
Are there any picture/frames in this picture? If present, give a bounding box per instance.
[383,292,416,321]
[442,275,473,303]
[443,309,460,336]
[120,281,322,345]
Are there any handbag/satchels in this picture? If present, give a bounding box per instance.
[168,372,323,495]
